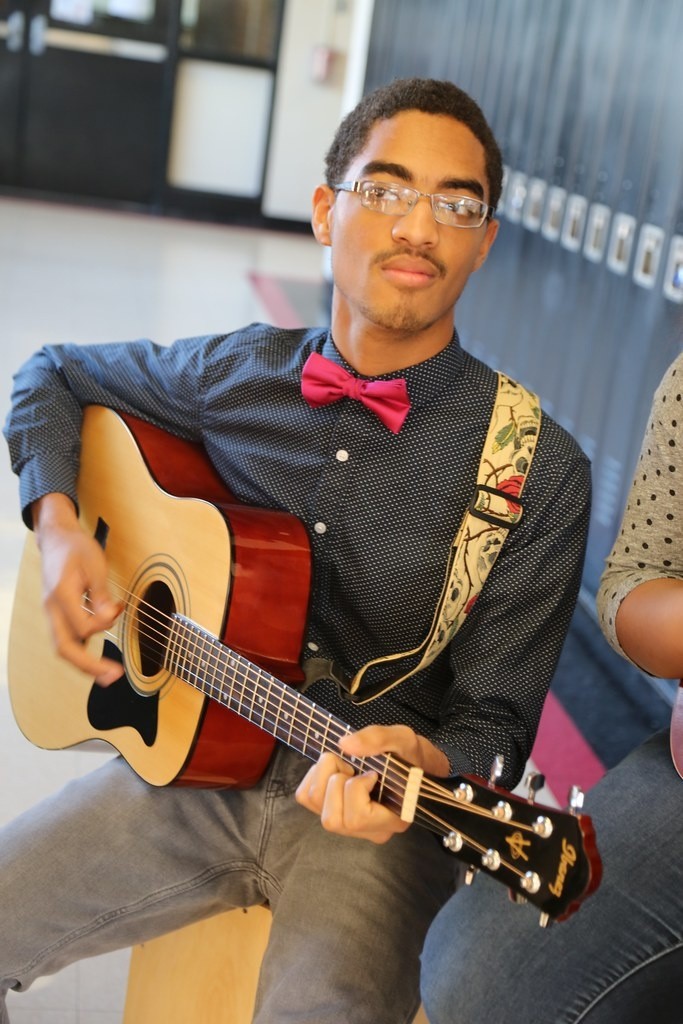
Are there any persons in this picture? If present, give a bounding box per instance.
[419,353,683,1024]
[0,75,593,1023]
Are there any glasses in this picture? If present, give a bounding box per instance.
[333,179,495,231]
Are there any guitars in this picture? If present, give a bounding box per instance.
[4,401,606,931]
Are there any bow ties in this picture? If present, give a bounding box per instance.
[301,352,412,434]
